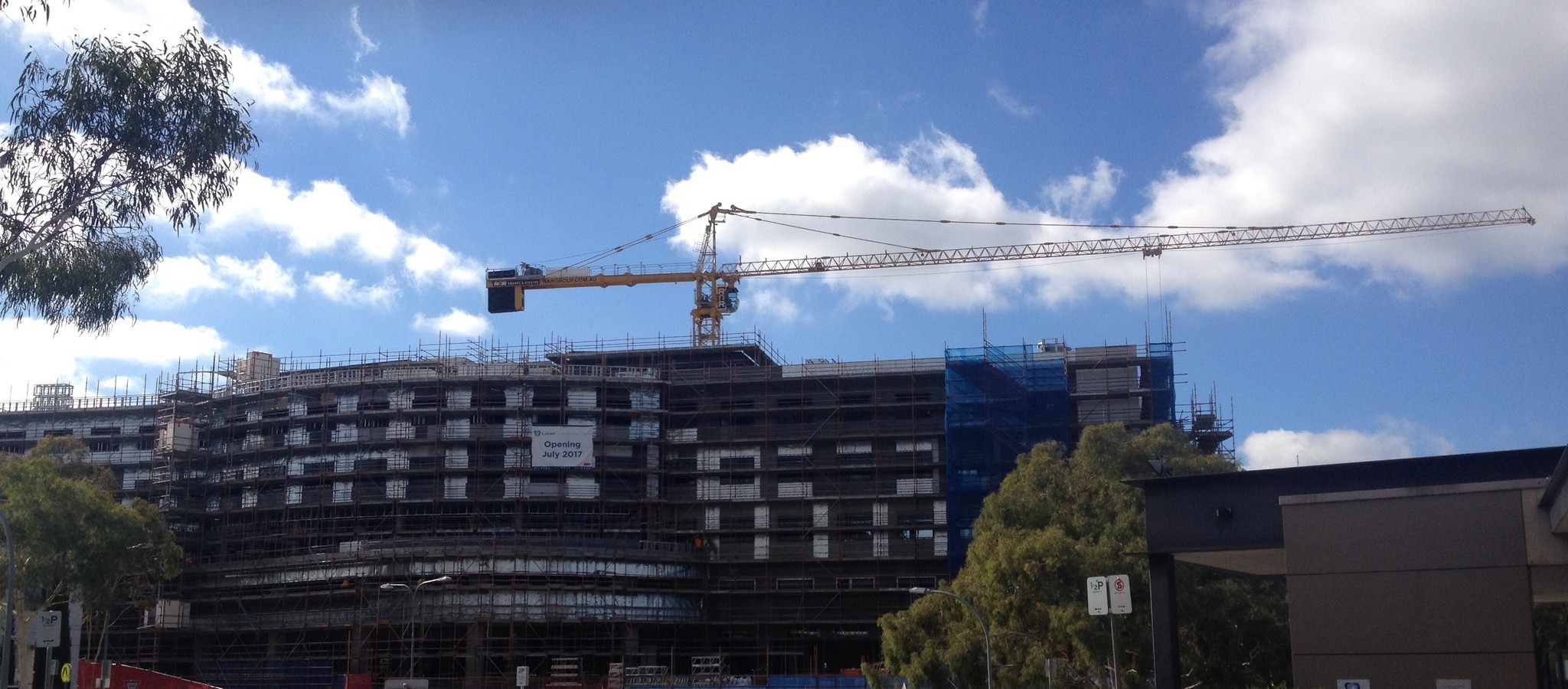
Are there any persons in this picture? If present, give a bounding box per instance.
[695,535,709,547]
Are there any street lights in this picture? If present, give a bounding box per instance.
[379,576,453,679]
[908,587,993,689]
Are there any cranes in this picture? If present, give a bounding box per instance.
[482,200,1539,348]
